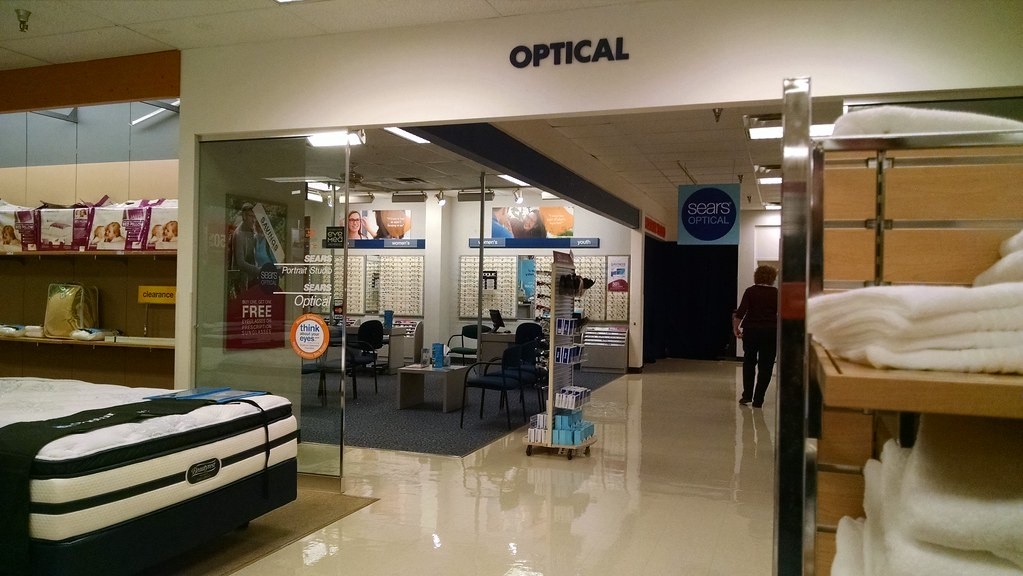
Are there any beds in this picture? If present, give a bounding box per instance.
[0,371,301,575]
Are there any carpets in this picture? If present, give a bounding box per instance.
[112,482,385,576]
[300,359,626,458]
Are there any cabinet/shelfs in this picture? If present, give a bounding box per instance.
[2,249,177,351]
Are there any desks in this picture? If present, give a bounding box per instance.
[479,330,518,371]
[399,364,469,412]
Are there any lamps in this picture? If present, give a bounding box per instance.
[307,183,560,206]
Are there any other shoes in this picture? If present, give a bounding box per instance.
[752,402,762,408]
[739,397,752,404]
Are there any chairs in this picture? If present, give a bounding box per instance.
[457,343,527,428]
[302,326,358,409]
[344,319,384,397]
[495,322,544,412]
[447,324,492,365]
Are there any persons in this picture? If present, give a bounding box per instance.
[348,211,405,239]
[232,203,263,292]
[492,208,547,239]
[2,225,21,245]
[734,266,778,408]
[91,222,125,243]
[150,221,178,242]
[75,209,88,218]
